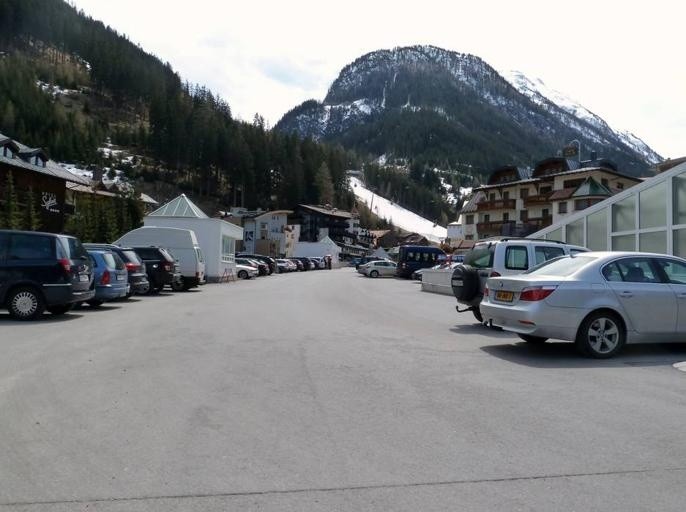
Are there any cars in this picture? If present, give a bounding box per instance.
[235,252,325,281]
[474,247,686,367]
[350,244,466,290]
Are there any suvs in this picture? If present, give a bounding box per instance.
[0,226,205,318]
[451,233,593,332]
